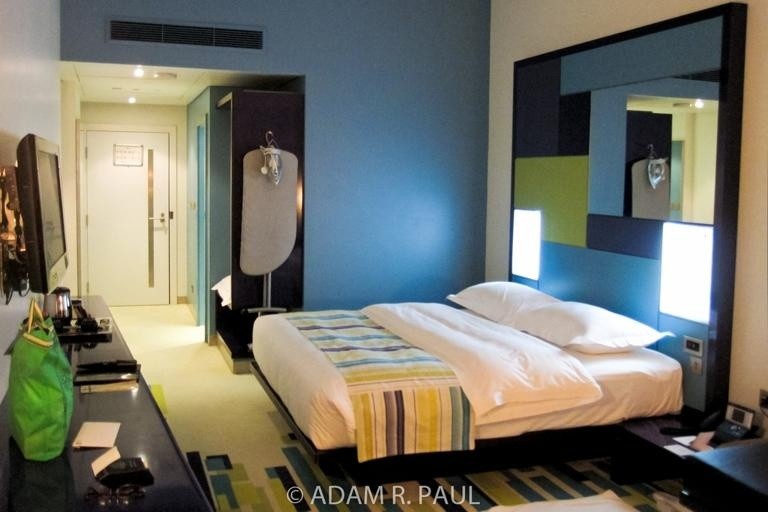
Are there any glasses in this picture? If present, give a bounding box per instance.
[84,484,147,501]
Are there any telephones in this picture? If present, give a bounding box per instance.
[700,408,723,430]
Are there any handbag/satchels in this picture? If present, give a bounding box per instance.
[10,301,74,463]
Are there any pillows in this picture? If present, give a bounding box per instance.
[446,281,677,354]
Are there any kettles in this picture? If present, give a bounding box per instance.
[41,286,72,326]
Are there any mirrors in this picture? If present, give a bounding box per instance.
[622,94,718,225]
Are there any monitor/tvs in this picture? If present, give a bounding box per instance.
[14,132,71,295]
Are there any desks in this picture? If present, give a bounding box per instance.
[0,294,212,511]
[618,416,695,487]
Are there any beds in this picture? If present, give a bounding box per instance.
[253,309,685,485]
